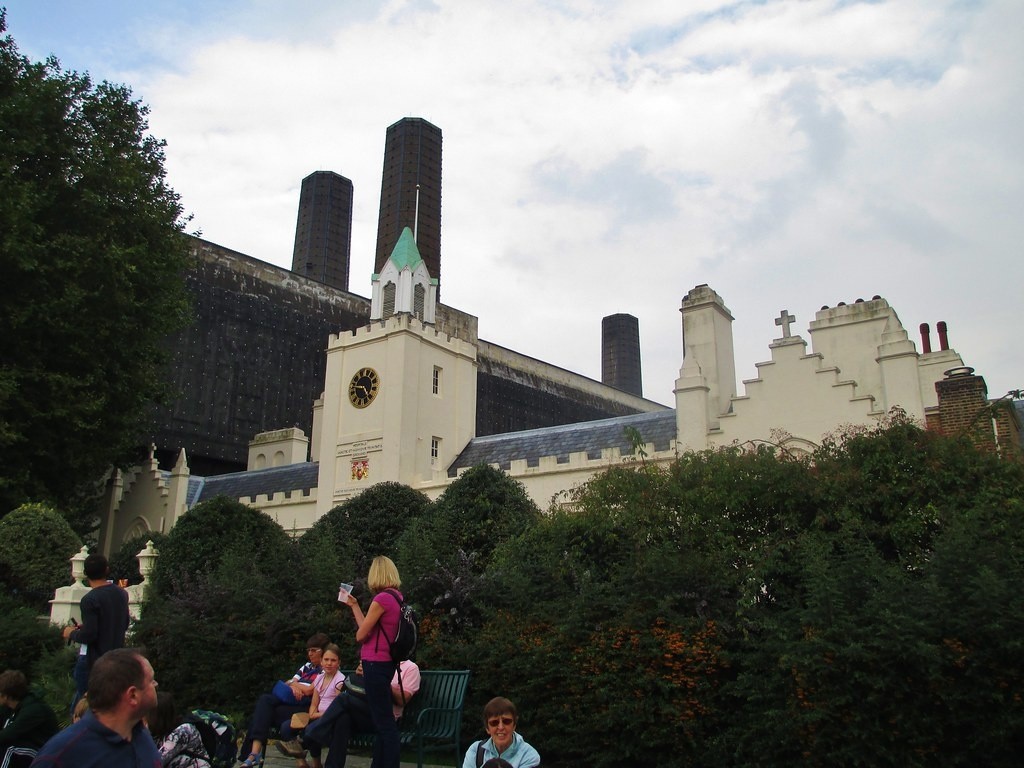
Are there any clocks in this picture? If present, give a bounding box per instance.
[348,367,380,408]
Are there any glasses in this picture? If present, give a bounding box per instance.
[489,719,512,726]
[308,649,316,652]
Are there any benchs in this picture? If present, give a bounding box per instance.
[258,669,470,768]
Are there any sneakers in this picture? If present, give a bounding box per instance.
[276,735,308,759]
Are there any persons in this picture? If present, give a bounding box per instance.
[0,552,210,768]
[236,634,421,768]
[462,697,541,768]
[339,555,404,768]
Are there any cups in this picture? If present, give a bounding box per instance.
[338,583,353,603]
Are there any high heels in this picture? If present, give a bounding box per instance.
[240,752,264,768]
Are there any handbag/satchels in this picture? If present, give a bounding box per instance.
[271,679,309,705]
[335,672,368,699]
[290,713,309,728]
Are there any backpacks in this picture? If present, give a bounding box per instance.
[366,590,419,661]
[168,709,238,768]
